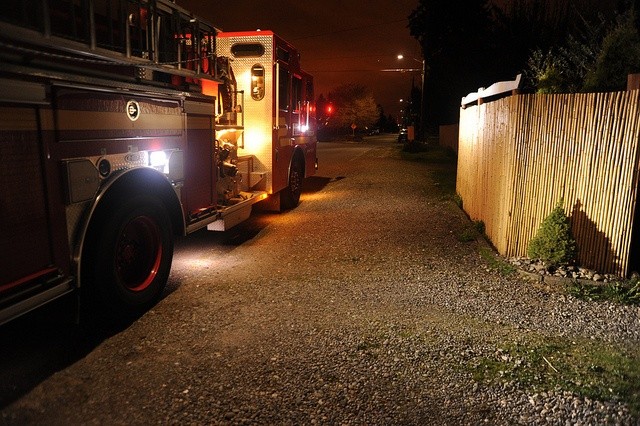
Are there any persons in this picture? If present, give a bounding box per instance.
[216,146,242,198]
[128,102,138,119]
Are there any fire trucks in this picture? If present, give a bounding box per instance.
[0,0,320,326]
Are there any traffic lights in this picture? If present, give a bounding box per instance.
[328,101,333,113]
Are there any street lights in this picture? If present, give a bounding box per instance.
[397,53,424,140]
